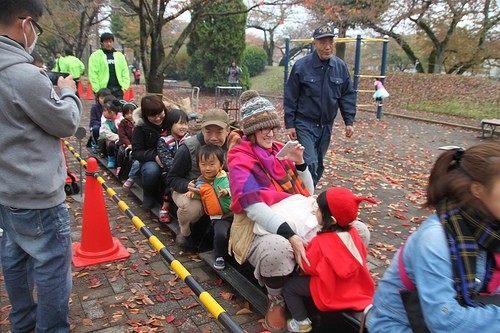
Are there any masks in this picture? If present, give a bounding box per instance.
[22,19,37,53]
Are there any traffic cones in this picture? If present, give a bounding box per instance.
[70,157,131,267]
[85,79,94,100]
[123,86,134,102]
[77,79,85,97]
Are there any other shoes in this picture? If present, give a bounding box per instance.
[285,318,312,332]
[264,293,286,332]
[213,256,225,270]
[159,208,171,222]
[117,167,121,175]
[108,157,116,169]
[91,142,97,154]
[123,181,133,188]
[96,148,105,158]
[175,231,190,246]
[142,196,156,210]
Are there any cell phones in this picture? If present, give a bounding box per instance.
[276,142,297,158]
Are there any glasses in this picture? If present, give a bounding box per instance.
[18,17,44,36]
[259,127,278,134]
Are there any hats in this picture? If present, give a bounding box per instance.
[326,187,378,230]
[312,26,335,39]
[239,90,282,134]
[201,108,230,128]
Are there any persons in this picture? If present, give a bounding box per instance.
[0,0,82,333]
[186,145,231,269]
[90,88,192,223]
[35,49,85,97]
[366,143,500,333]
[167,109,230,247]
[372,78,389,100]
[284,25,357,187]
[227,90,370,333]
[88,33,130,99]
[283,187,378,333]
[227,60,242,95]
[134,69,141,85]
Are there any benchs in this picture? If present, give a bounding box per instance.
[479,118,500,139]
